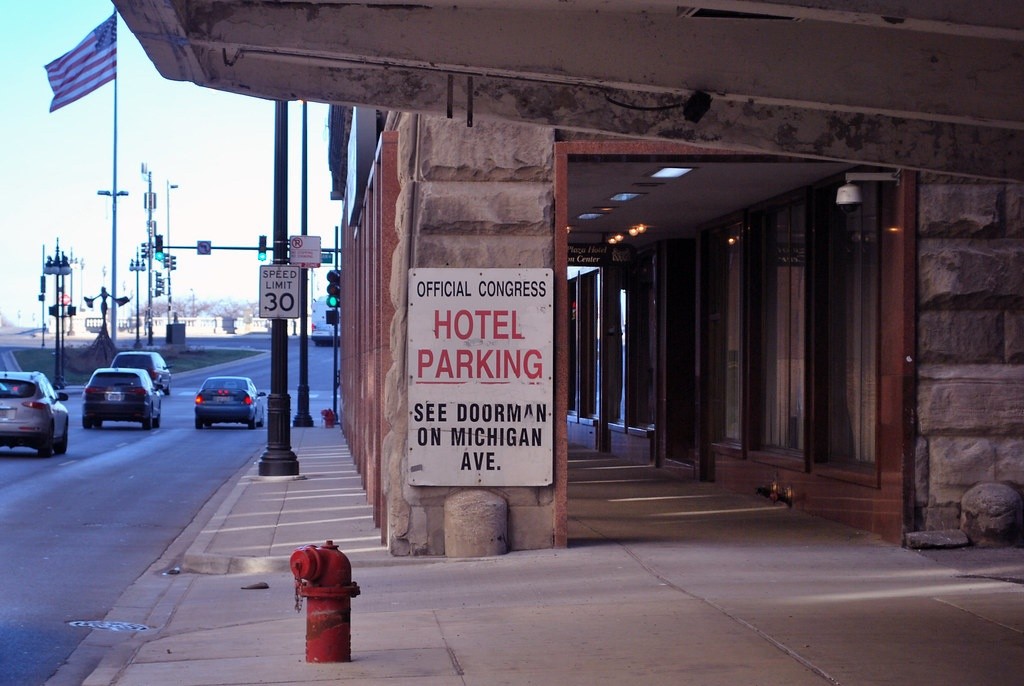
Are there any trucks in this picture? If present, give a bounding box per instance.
[311,296,341,347]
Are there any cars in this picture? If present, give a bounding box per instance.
[194,376,267,429]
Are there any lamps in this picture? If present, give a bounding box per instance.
[605,92,713,124]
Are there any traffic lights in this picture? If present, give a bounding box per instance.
[326,270,341,307]
[156,272,163,288]
[164,253,170,268]
[259,235,266,261]
[156,235,163,260]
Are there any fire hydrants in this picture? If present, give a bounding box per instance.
[290,540,360,663]
[321,408,335,428]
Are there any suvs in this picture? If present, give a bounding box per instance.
[81,367,164,430]
[110,351,173,395]
[0,371,69,458]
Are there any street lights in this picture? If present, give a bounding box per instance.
[97,190,129,346]
[129,247,146,348]
[167,182,178,322]
[43,239,72,389]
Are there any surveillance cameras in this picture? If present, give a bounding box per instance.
[835,184,863,211]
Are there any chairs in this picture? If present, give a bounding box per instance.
[224,381,238,388]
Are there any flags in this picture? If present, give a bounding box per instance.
[44,12,117,113]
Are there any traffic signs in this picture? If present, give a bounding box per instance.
[171,255,176,270]
[259,265,300,318]
